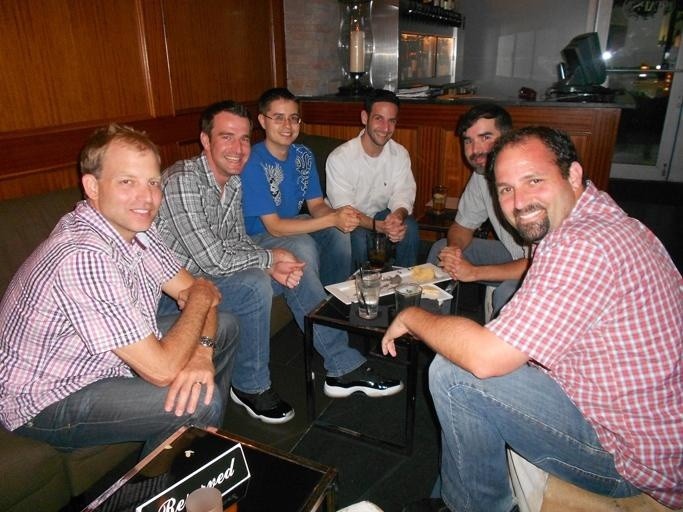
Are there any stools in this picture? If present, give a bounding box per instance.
[479,286,683,512]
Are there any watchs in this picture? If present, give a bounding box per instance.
[197,336,217,357]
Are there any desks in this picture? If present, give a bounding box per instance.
[544,89,638,108]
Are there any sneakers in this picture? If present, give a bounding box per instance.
[323,359,406,399]
[229,383,295,424]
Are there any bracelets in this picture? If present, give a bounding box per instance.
[373,218,375,233]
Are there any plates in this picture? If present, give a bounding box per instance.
[324,261,452,308]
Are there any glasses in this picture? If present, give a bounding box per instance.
[262,113,302,125]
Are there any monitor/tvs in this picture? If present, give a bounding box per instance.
[562,46,593,87]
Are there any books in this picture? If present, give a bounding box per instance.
[395,84,445,100]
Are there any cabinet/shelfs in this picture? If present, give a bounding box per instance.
[399,32,453,83]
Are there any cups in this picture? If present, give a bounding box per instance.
[367,231,387,270]
[394,281,423,314]
[355,271,382,320]
[184,486,223,512]
[431,185,449,216]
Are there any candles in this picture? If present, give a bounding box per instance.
[349,27,365,72]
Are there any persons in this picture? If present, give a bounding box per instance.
[381,125,683,512]
[237,84,362,297]
[427,102,543,322]
[0,122,240,469]
[148,99,406,424]
[322,87,420,276]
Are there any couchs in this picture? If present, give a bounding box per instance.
[1,137,347,510]
